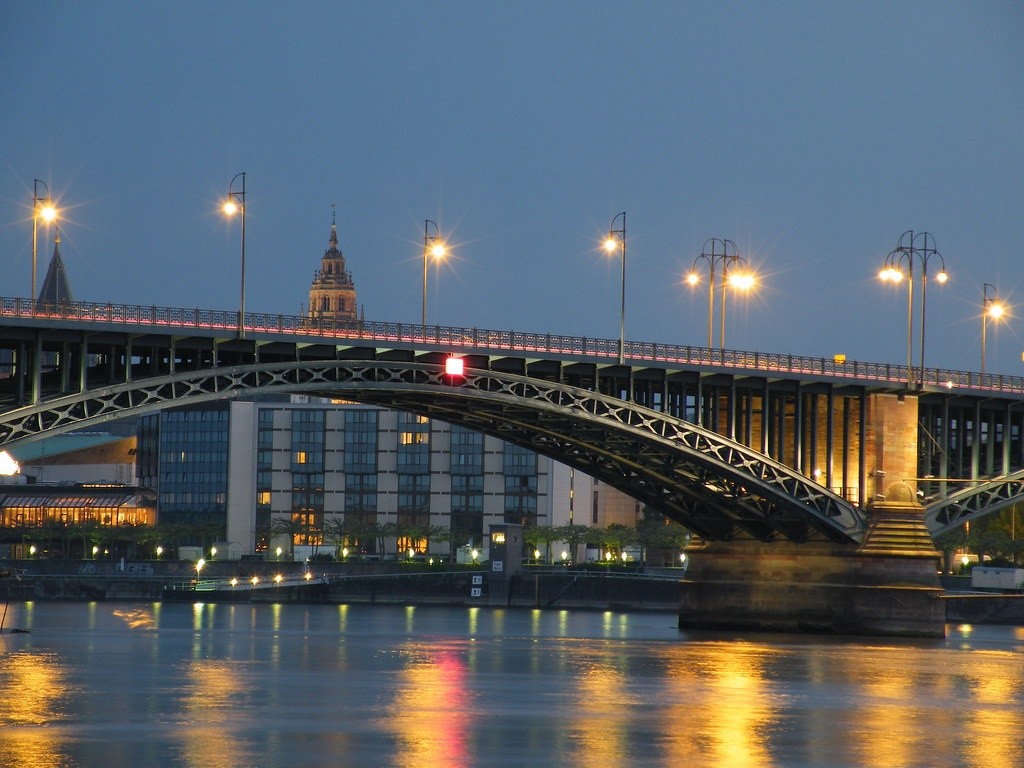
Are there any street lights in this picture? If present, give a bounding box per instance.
[687,238,725,361]
[981,283,1005,375]
[606,212,627,365]
[422,219,446,333]
[223,172,248,339]
[720,239,755,360]
[880,230,948,385]
[30,178,56,299]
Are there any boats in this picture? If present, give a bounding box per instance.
[191,575,330,604]
